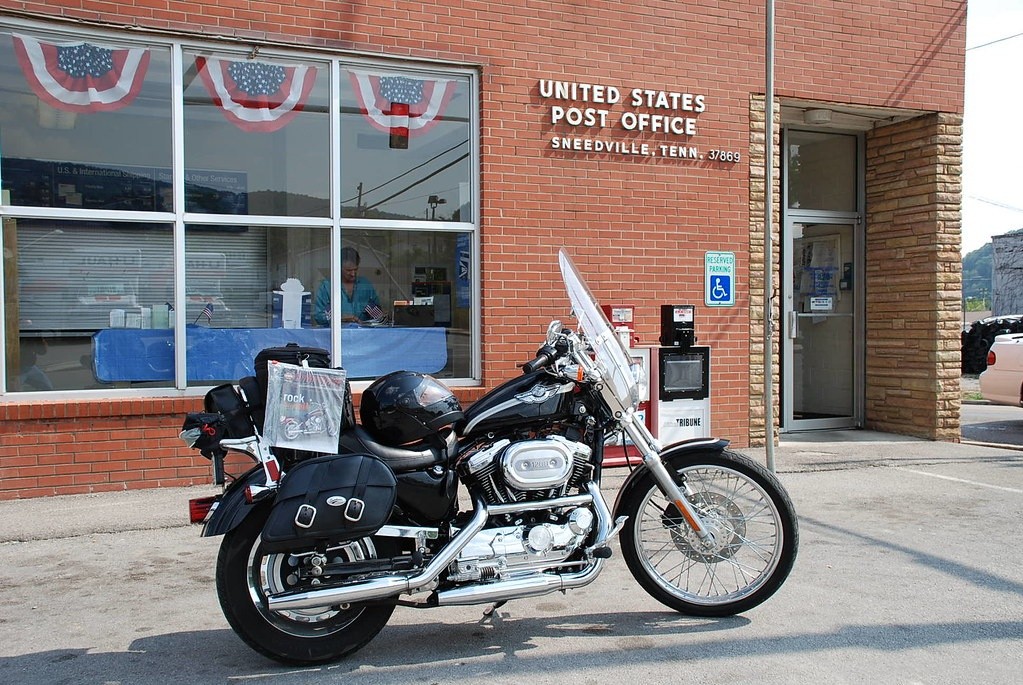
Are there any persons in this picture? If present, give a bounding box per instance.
[311,246,383,326]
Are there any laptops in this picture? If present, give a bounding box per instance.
[388,305,436,327]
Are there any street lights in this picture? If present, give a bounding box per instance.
[428,195,446,258]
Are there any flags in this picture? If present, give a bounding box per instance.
[364,301,382,320]
[203,303,213,324]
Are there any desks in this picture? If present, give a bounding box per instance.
[94,325,446,388]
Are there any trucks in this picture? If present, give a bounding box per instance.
[144,249,230,326]
[63,247,140,326]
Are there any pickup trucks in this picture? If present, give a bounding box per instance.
[978,332,1023,407]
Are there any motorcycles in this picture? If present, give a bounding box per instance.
[176,245,801,667]
